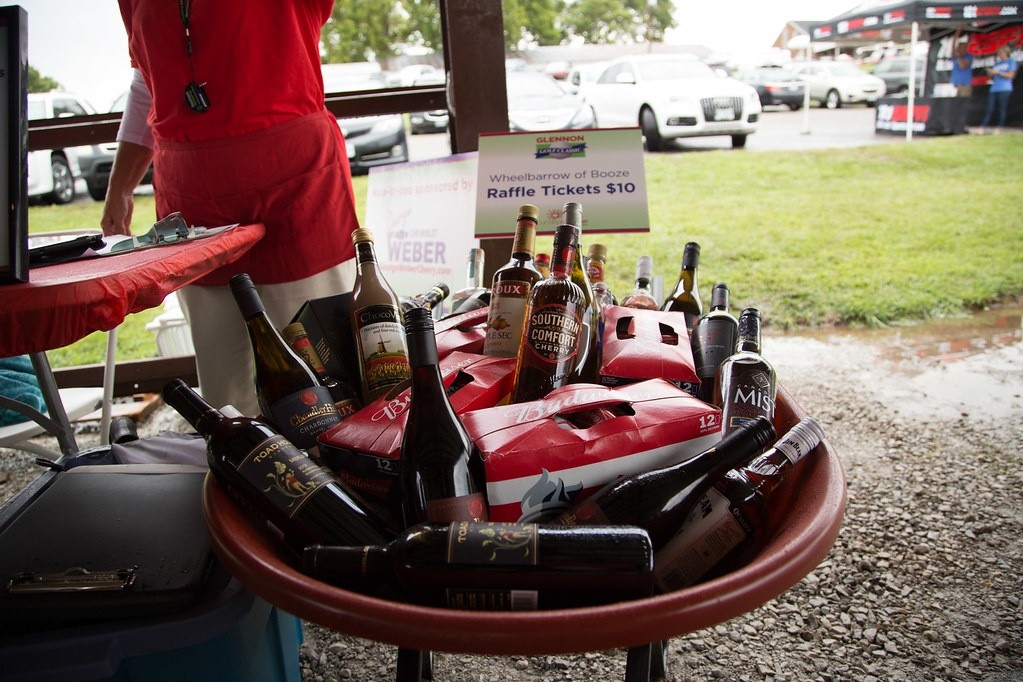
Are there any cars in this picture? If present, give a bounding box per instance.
[28,40,942,213]
[502,70,599,134]
[587,54,764,151]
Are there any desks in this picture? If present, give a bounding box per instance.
[877,97,970,138]
[0,224,263,463]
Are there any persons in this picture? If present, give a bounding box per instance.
[100,0,362,421]
[976,46,1016,135]
[951,25,974,134]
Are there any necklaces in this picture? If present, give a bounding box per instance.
[176,0,212,116]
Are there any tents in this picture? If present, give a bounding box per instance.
[803,0,1023,144]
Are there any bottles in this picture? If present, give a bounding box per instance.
[158,199,827,609]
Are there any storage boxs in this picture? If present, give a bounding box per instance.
[1,431,306,681]
